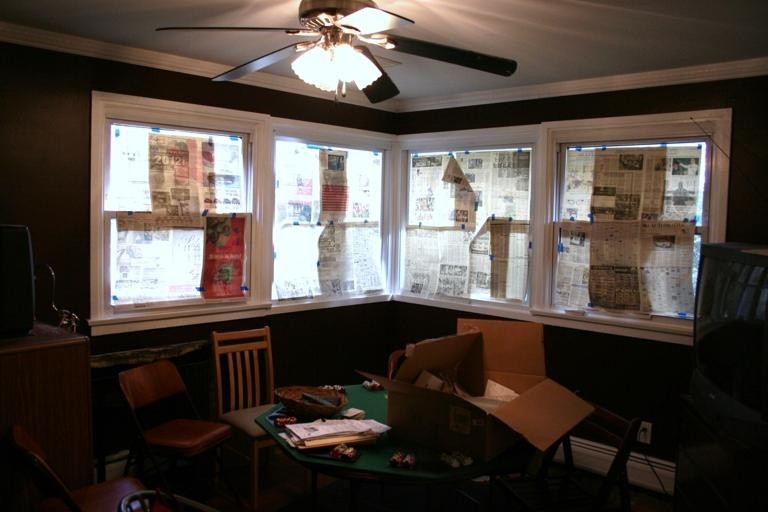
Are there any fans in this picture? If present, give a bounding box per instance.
[149,1,517,104]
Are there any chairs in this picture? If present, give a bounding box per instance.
[517,388,640,510]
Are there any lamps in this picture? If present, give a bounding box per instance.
[288,23,383,94]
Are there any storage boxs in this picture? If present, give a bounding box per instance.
[386,315,595,461]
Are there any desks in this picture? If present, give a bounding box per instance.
[256,380,530,512]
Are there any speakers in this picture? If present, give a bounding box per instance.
[2,225,34,339]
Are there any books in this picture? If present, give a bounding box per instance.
[277,418,393,450]
